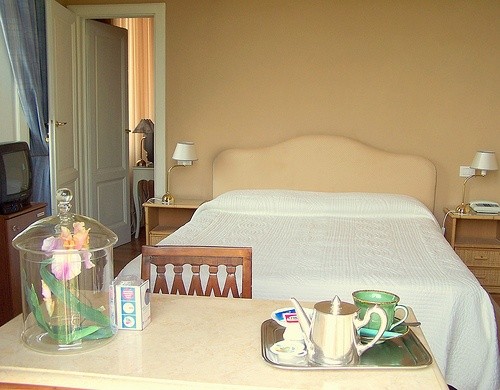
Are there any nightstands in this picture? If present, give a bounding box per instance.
[142,198,210,246]
[444,208,500,294]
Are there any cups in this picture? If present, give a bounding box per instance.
[351,289,408,331]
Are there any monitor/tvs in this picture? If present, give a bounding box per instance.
[0,141,34,213]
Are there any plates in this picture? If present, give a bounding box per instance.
[360,317,409,344]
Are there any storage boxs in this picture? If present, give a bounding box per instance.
[110,275,152,332]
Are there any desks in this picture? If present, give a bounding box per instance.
[0,288,451,390]
[130,166,154,238]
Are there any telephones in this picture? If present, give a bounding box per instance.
[468,200,500,213]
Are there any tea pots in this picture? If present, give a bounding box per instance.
[289,295,388,367]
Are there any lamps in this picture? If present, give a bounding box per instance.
[162,140,199,204]
[133,119,155,166]
[455,149,498,213]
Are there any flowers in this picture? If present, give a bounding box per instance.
[22,222,113,345]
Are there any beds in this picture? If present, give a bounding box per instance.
[113,134,500,390]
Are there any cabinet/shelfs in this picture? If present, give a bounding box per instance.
[0,200,49,327]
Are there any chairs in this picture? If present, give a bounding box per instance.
[141,245,253,300]
